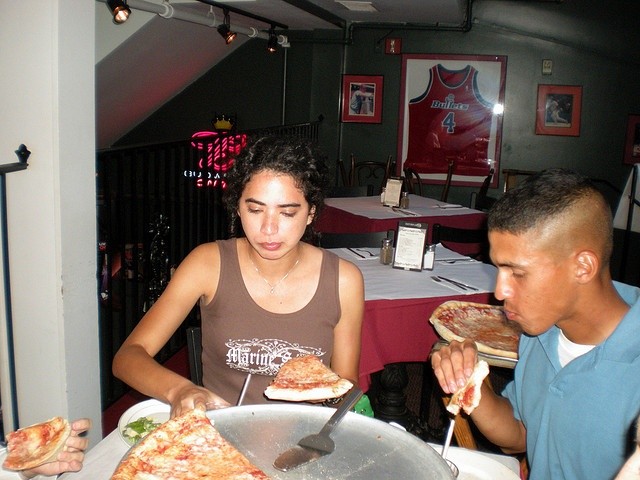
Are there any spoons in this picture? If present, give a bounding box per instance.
[430,276,444,283]
[356,248,378,257]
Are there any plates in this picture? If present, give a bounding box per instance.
[118,399,171,450]
[113,404,454,476]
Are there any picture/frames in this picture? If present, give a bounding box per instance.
[340,74,383,125]
[624,115,639,165]
[385,37,401,55]
[395,54,507,189]
[534,84,582,137]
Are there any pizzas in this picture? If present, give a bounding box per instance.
[1,415,71,471]
[429,300,521,359]
[264,353,354,402]
[107,408,273,480]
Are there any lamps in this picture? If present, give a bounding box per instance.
[217,9,238,45]
[107,2,132,25]
[266,24,277,53]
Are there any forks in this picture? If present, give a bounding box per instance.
[437,204,462,208]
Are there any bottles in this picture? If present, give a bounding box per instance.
[380,187,386,204]
[423,243,436,270]
[402,192,410,210]
[381,238,394,265]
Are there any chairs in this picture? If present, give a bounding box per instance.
[186,326,202,387]
[441,159,455,202]
[475,167,494,210]
[404,167,422,195]
[338,154,395,197]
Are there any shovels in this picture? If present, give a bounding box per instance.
[273,387,364,473]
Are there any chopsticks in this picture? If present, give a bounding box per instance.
[347,246,365,259]
[438,275,479,292]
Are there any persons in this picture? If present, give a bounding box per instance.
[348,84,372,115]
[431,168,640,480]
[0,419,91,480]
[111,135,367,419]
